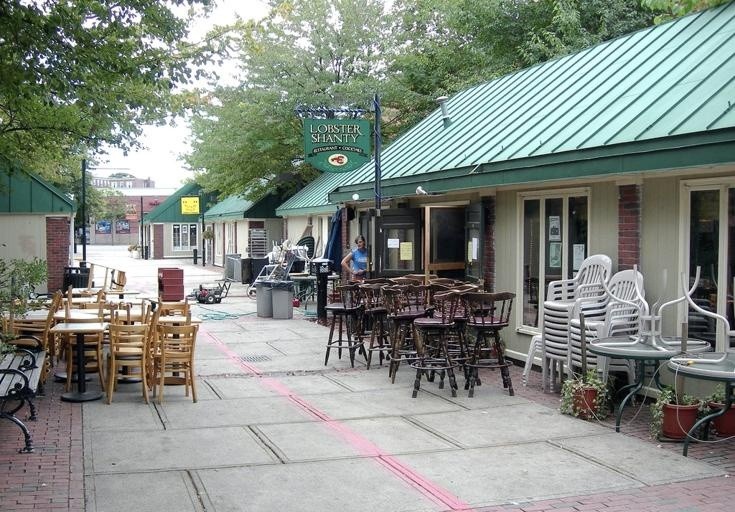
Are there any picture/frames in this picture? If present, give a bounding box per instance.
[289,276,340,310]
[589,263,735,456]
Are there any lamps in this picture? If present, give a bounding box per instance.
[323,274,516,397]
[0,284,199,405]
[521,254,648,415]
[74,258,127,293]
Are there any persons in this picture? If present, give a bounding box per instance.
[340,235,368,336]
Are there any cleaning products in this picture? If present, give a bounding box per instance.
[195,279,231,304]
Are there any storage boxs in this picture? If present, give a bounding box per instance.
[0,334,48,454]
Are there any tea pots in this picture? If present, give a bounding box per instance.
[467,227,481,278]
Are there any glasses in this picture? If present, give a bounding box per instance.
[416,186,428,195]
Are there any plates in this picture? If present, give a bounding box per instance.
[158,268,184,302]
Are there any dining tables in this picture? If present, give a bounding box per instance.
[559,369,615,422]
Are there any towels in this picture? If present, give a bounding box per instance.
[255,281,272,318]
[272,281,295,319]
[63,267,90,297]
